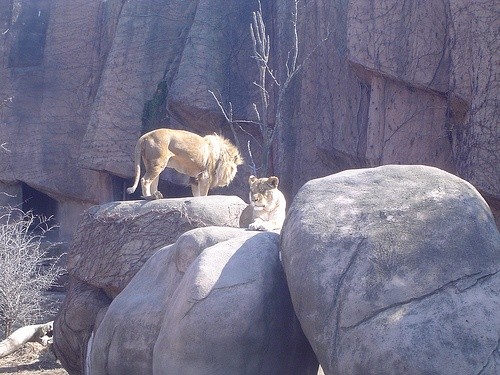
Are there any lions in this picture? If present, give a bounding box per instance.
[248,174,288,236]
[126,128,245,199]
